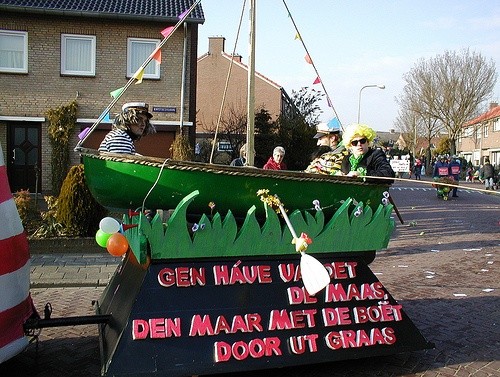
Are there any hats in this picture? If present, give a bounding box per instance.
[122,103,153,119]
[313,123,340,138]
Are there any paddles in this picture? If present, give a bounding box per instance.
[277,198,332,296]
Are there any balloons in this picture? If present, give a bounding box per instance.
[96,217,129,257]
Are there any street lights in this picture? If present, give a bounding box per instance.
[358,84,385,124]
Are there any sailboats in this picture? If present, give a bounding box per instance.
[73,0,395,222]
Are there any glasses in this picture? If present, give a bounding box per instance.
[351,138,367,146]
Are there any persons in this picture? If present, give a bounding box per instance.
[479,158,495,190]
[342,125,398,179]
[432,155,462,198]
[305,123,350,176]
[230,143,256,166]
[263,146,288,170]
[98,102,158,155]
[411,159,422,180]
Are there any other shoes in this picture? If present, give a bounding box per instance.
[452,195,459,197]
